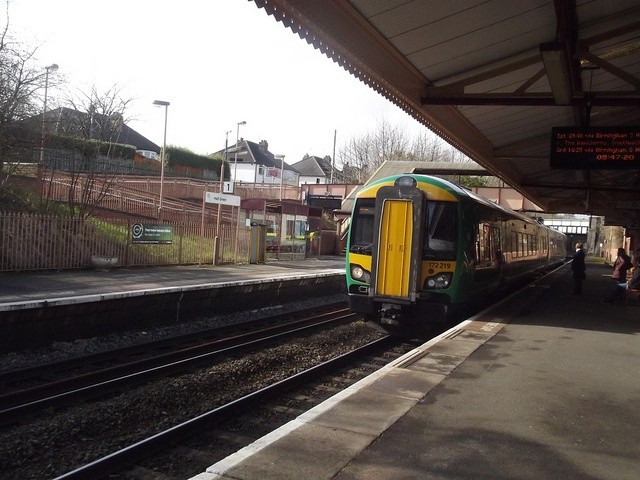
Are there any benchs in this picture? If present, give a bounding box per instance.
[625,288,640,305]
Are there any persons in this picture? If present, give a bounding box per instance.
[611,248,630,300]
[571,243,585,295]
[600,253,640,304]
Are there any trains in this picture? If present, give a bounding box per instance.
[345,174,568,339]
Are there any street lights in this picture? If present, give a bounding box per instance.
[276,154,285,202]
[40,64,58,165]
[234,121,246,187]
[153,100,170,221]
